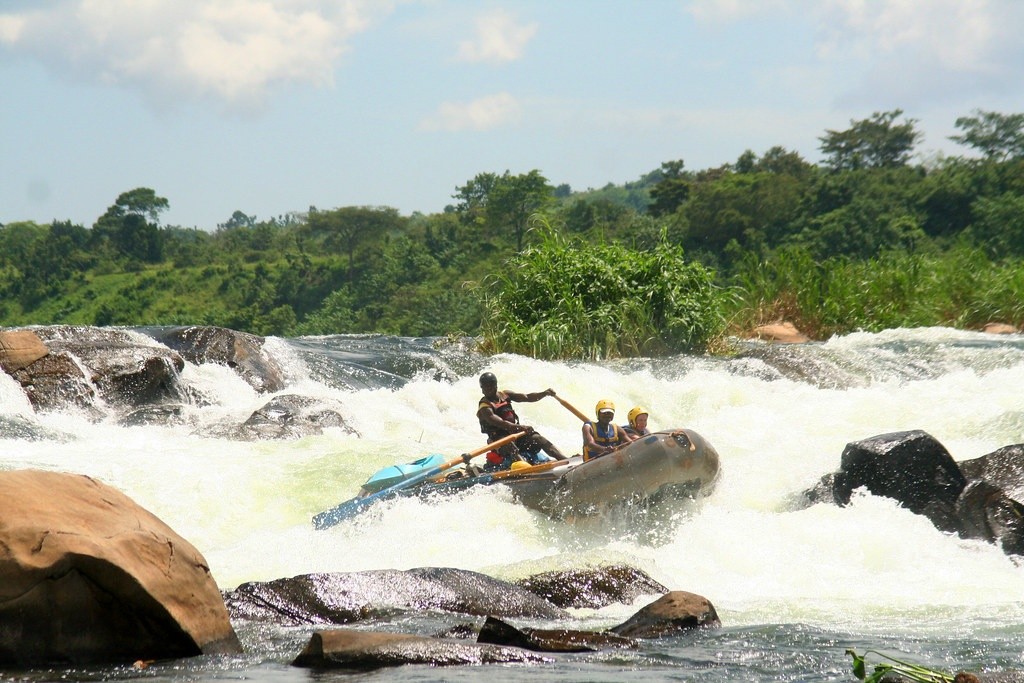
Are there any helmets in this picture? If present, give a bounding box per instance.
[480,372,497,387]
[595,401,615,420]
[628,407,648,428]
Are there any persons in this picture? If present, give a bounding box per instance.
[620,407,651,441]
[582,400,633,462]
[477,372,567,466]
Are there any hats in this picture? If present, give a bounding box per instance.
[600,408,615,414]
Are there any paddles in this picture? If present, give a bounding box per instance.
[397,459,569,498]
[311,430,526,531]
[551,391,592,423]
